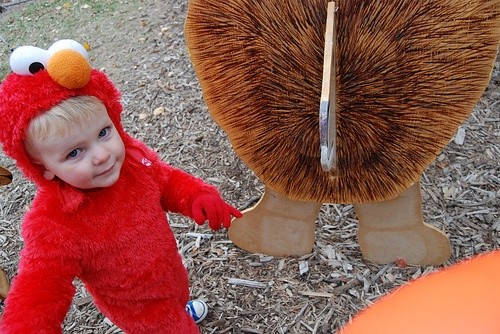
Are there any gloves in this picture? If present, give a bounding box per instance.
[192,193,243,230]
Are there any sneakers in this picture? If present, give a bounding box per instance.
[185,300,208,323]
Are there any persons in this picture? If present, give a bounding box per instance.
[0,38,244,334]
